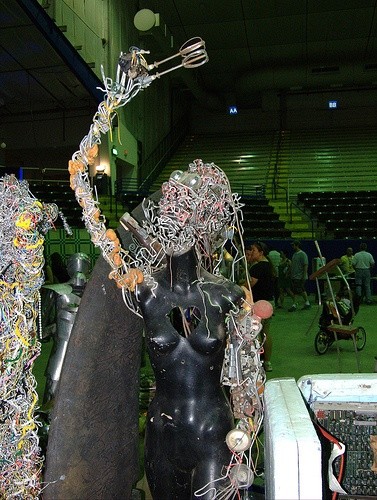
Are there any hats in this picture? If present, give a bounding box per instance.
[290,240,301,245]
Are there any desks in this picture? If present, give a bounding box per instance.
[310,276,377,304]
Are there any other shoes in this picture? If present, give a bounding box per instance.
[264,360,273,372]
[301,304,311,310]
[288,307,296,312]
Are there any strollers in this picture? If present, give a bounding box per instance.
[314,282,367,355]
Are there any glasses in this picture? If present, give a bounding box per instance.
[169,169,202,192]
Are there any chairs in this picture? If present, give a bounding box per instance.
[113,190,292,241]
[0,145,81,182]
[29,182,110,229]
[296,190,377,240]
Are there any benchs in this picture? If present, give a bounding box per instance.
[93,126,377,241]
